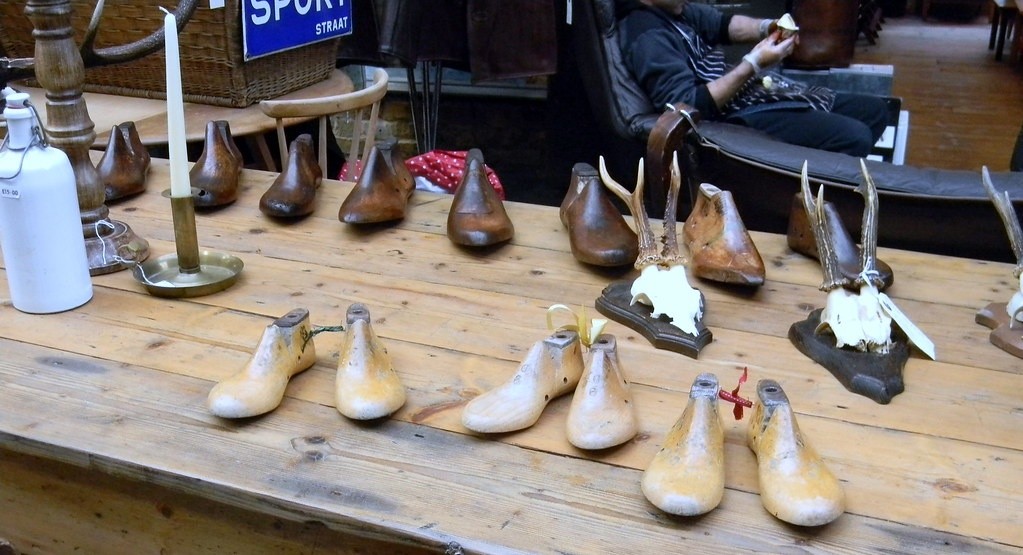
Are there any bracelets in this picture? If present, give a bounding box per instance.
[760,19,774,39]
[742,55,759,74]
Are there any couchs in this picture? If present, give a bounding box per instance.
[592,0,1023,261]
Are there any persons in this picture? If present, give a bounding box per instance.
[615,0,888,159]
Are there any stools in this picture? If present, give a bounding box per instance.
[989,0,1023,60]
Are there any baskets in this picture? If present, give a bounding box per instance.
[0,0,340,107]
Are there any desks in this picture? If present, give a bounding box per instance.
[0,146,1023,555]
[0,68,353,175]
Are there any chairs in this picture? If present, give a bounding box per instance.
[260,66,388,183]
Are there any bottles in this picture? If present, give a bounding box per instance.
[0,92,94,314]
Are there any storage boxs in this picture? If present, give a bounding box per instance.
[0,0,341,108]
